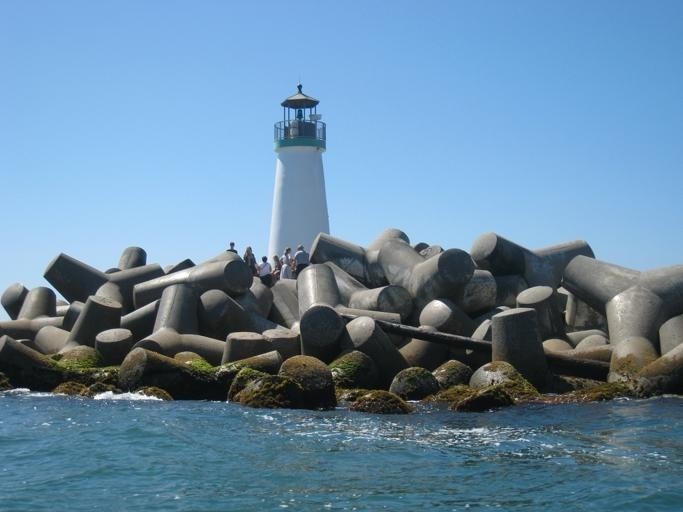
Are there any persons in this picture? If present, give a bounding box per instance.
[226,240,309,287]
